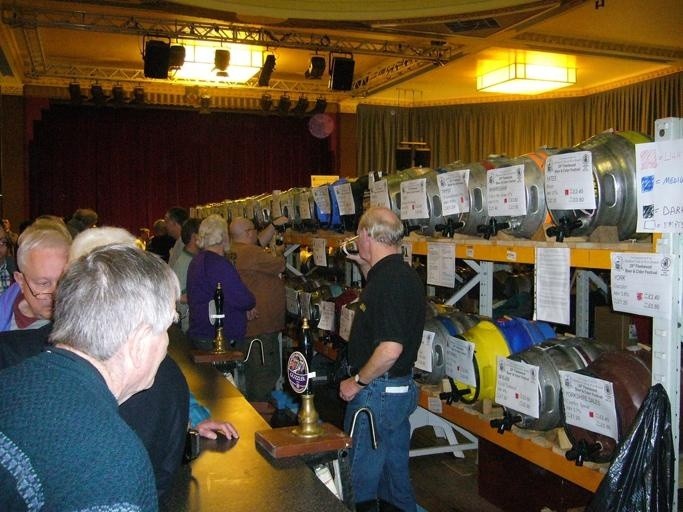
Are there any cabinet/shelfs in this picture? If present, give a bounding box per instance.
[280,230,655,493]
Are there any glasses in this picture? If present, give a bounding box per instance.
[18,269,54,302]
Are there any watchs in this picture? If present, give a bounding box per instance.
[354,372,371,390]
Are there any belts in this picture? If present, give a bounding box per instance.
[343,366,412,382]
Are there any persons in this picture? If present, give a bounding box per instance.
[1,244,183,512]
[337,205,427,510]
[1,202,291,401]
[1,225,239,511]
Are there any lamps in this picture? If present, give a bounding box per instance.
[477,64,576,96]
[260,93,327,115]
[304,50,355,91]
[68,78,145,106]
[141,33,170,78]
